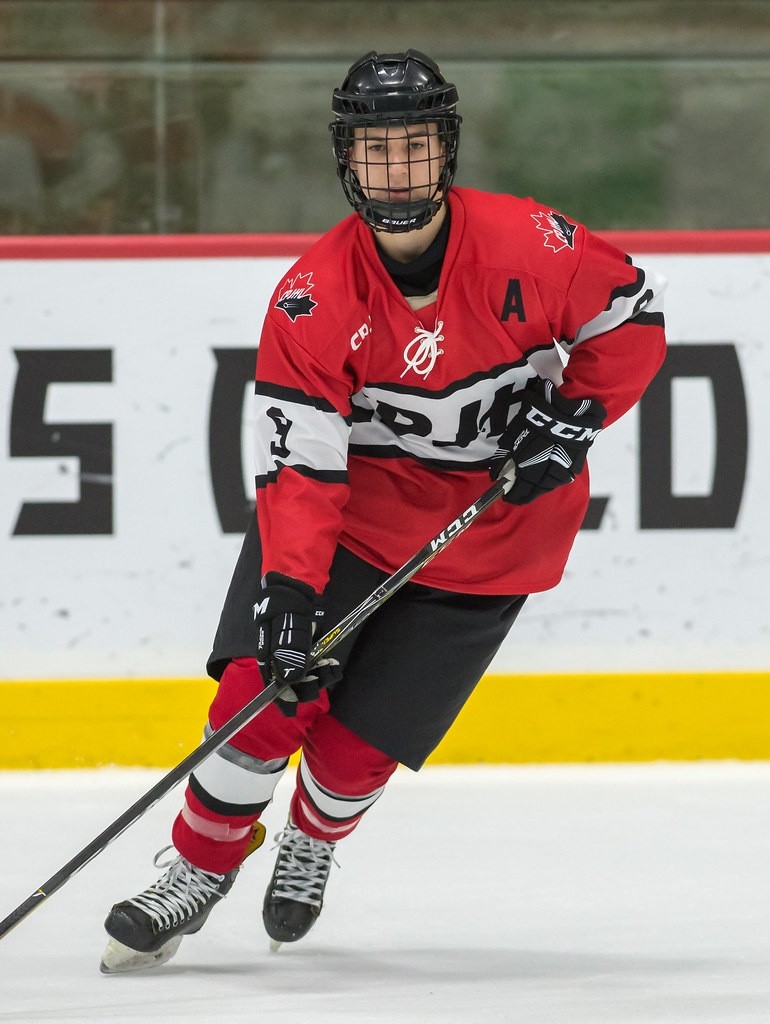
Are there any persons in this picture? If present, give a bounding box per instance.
[101,48,667,974]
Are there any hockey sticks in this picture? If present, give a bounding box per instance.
[1,465,507,940]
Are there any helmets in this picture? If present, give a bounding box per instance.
[329,49,464,235]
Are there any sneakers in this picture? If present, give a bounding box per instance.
[262,816,336,952]
[99,859,239,973]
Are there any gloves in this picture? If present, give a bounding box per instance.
[253,571,344,718]
[488,378,607,506]
